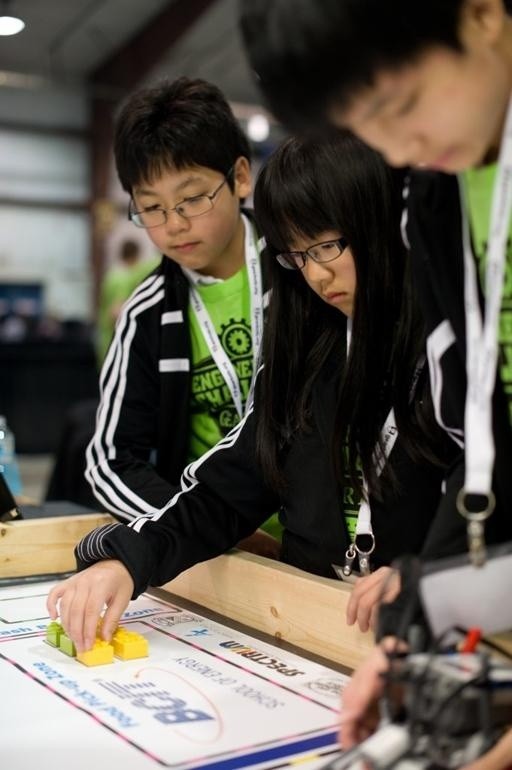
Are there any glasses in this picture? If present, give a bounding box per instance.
[272,236,349,271]
[128,167,233,228]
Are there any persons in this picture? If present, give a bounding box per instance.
[77,71,304,567]
[44,123,426,657]
[235,1,512,633]
[335,632,512,770]
